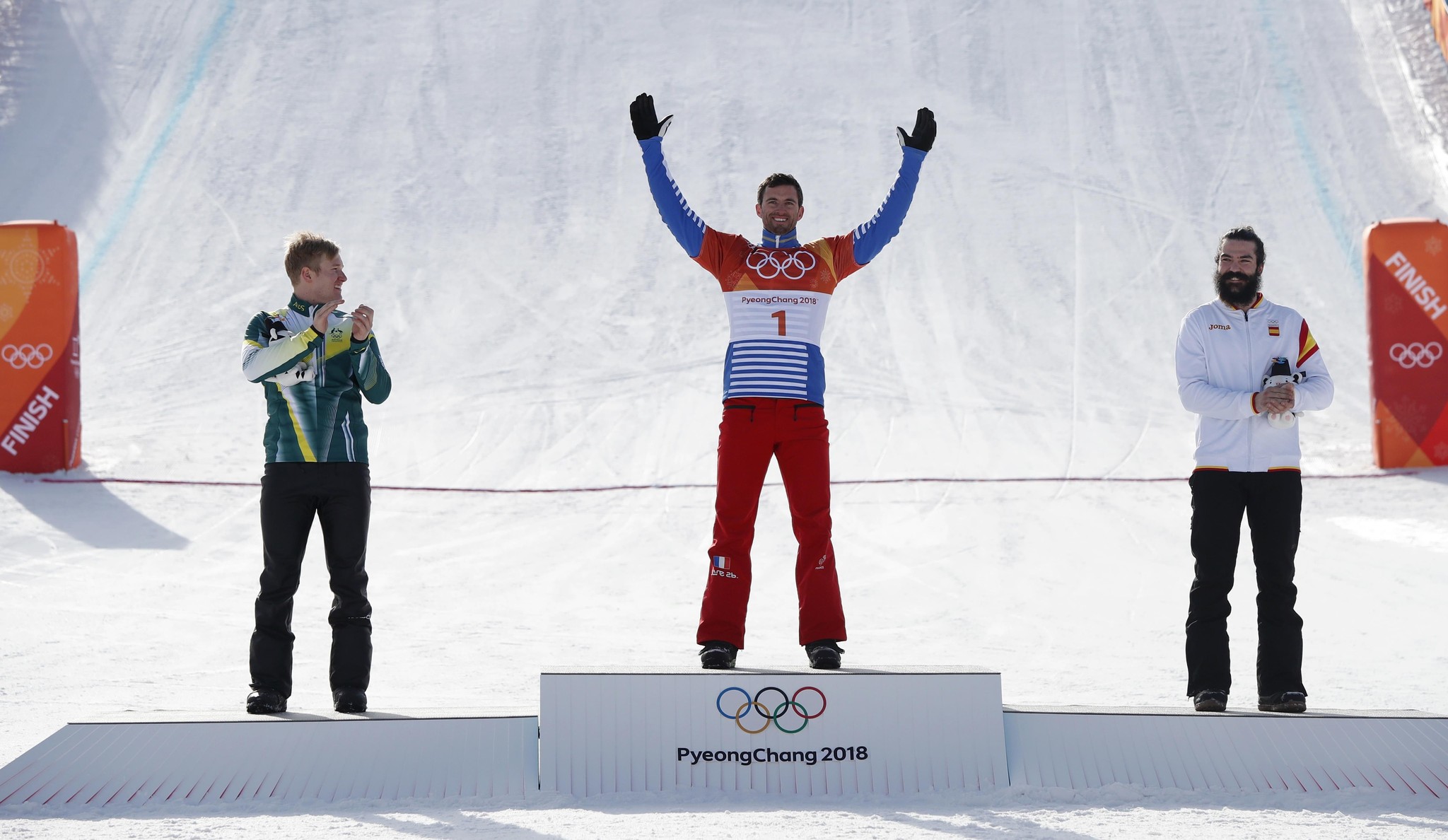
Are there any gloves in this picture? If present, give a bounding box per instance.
[896,107,937,152]
[630,93,674,141]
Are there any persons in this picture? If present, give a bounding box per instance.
[241,233,392,714]
[630,92,936,672]
[1178,229,1333,712]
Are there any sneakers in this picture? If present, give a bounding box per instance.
[332,686,367,713]
[246,686,287,714]
[698,640,739,670]
[1193,688,1227,712]
[805,638,845,669]
[1258,692,1306,713]
